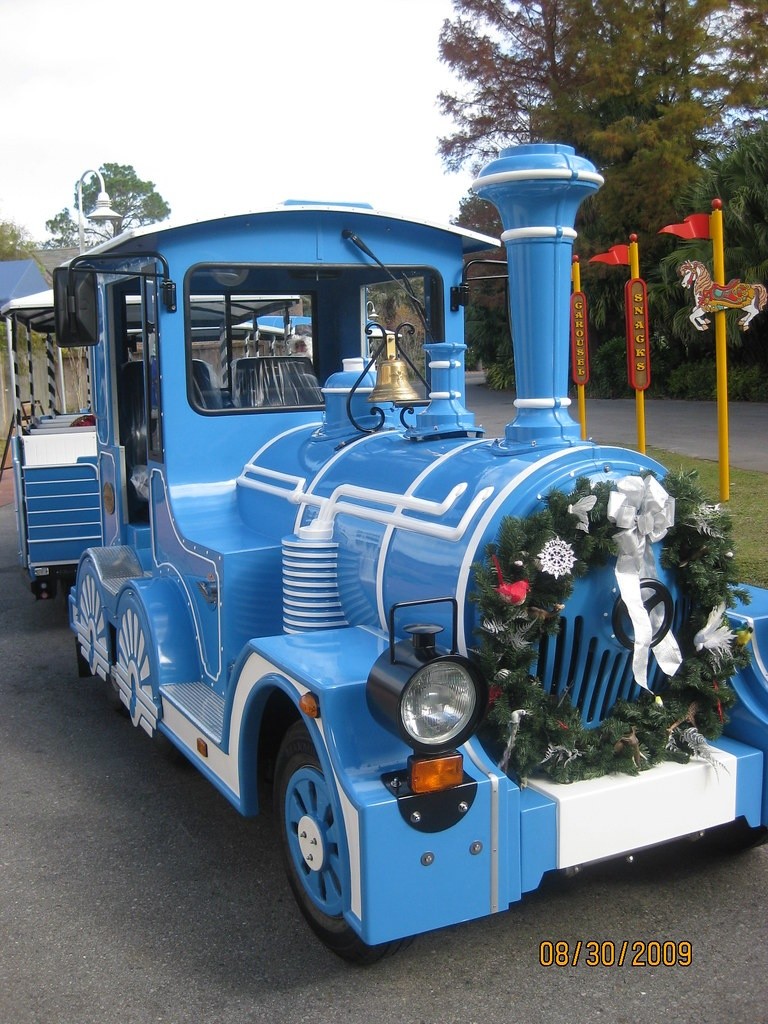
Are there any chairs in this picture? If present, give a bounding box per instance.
[230,354,323,409]
[120,358,224,467]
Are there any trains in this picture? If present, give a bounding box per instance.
[9,141,767,967]
